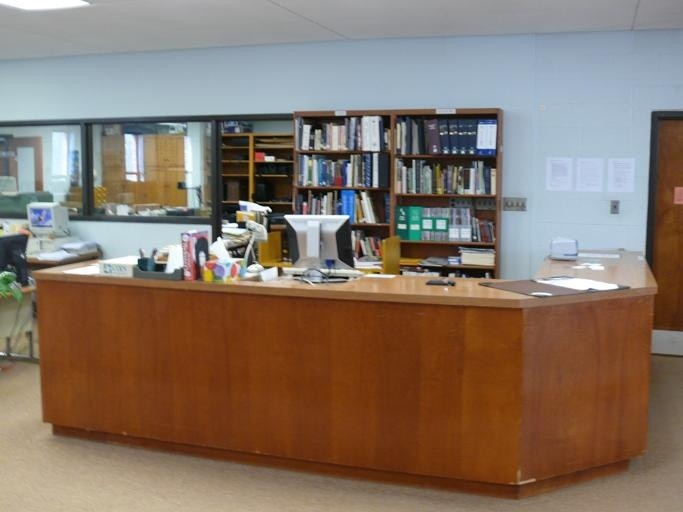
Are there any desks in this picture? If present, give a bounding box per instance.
[0,247,100,365]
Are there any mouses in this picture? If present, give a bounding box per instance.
[248,263,264,272]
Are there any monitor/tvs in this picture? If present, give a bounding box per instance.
[26,201,70,237]
[282,213,355,282]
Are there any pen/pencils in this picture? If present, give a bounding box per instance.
[139,247,144,258]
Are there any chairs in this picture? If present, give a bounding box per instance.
[257,230,293,268]
[117,192,160,211]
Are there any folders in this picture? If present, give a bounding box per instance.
[423,118,497,155]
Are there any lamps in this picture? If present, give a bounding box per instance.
[240,219,268,262]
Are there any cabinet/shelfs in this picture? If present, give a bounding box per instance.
[219,132,294,216]
[102,132,187,206]
[292,109,503,278]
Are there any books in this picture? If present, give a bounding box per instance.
[294,114,391,269]
[394,114,496,278]
[181,226,208,279]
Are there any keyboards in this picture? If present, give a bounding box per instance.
[284,266,364,277]
